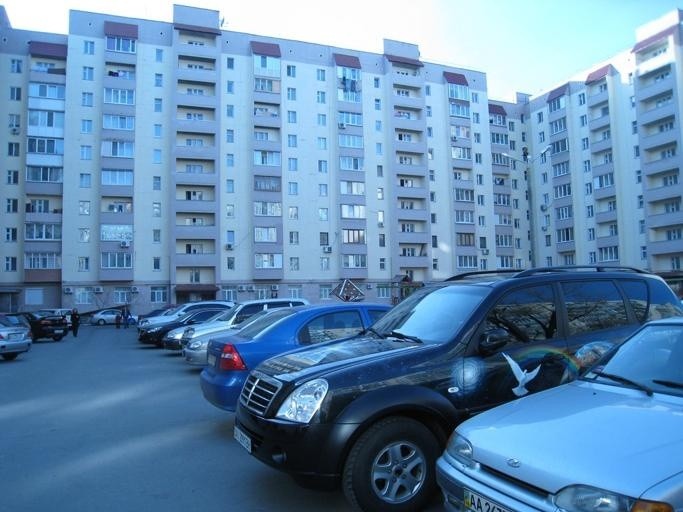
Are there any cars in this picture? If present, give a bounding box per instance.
[0,312,32,340]
[430,313,683,511]
[0,315,32,363]
[90,308,138,326]
[199,301,396,417]
[8,310,69,342]
[180,306,292,369]
[133,298,233,353]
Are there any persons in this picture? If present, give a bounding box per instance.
[122,306,133,329]
[70,307,81,337]
[115,314,121,327]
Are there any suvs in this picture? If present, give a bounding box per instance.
[227,263,683,510]
[180,298,314,349]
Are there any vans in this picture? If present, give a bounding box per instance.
[37,307,73,330]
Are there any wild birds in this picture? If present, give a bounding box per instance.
[501,351,541,397]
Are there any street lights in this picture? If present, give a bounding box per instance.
[501,140,555,267]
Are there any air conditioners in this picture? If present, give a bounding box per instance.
[238,285,255,291]
[226,244,234,250]
[94,286,103,293]
[121,240,129,246]
[131,285,138,293]
[324,247,333,252]
[271,285,279,291]
[482,249,489,255]
[367,284,372,288]
[339,123,346,129]
[64,287,72,293]
